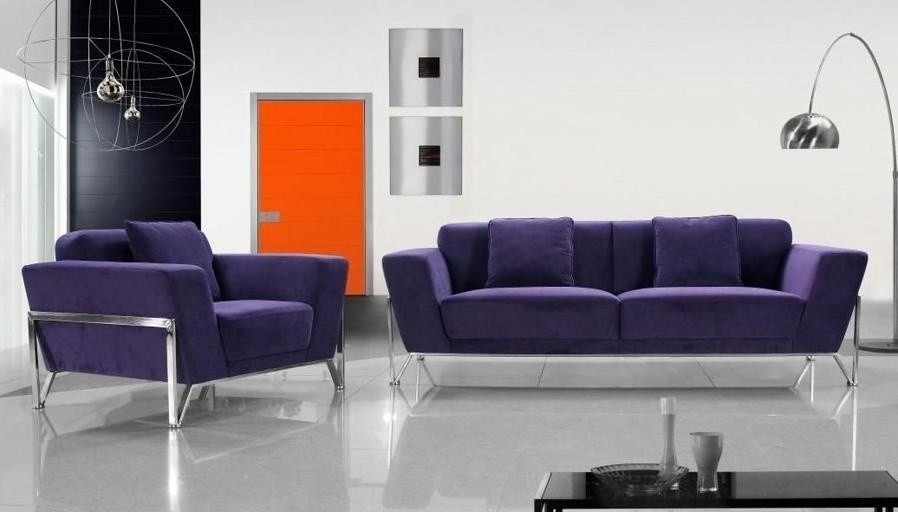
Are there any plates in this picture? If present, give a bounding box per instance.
[590,462,688,495]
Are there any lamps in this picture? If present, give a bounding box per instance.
[16,0,196,153]
[781,33,897,354]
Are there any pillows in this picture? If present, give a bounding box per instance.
[652,214,741,287]
[486,218,575,287]
[125,223,220,302]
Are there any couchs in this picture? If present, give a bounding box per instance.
[380,219,868,420]
[22,228,349,429]
